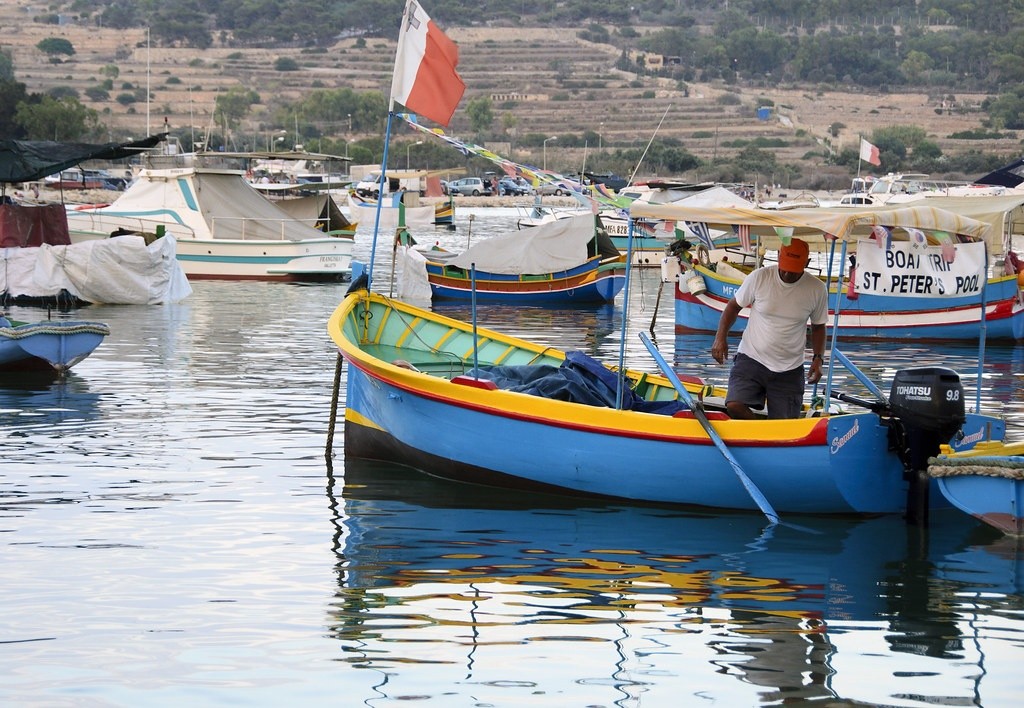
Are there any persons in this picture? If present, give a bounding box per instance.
[712,237,829,420]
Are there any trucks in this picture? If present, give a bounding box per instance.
[356,172,426,201]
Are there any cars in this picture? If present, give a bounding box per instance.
[449,175,571,196]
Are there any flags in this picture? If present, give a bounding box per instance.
[859,139,881,166]
[391,0,466,128]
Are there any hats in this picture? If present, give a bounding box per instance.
[778,237,809,273]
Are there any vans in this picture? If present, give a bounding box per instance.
[45,168,114,185]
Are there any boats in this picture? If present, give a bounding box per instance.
[327,0,1024,537]
[0,194,193,314]
[0,311,111,371]
[66,118,357,282]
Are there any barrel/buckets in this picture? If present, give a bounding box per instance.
[686,276,707,296]
[679,270,696,293]
[661,256,681,283]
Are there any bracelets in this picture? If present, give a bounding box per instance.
[812,354,824,365]
